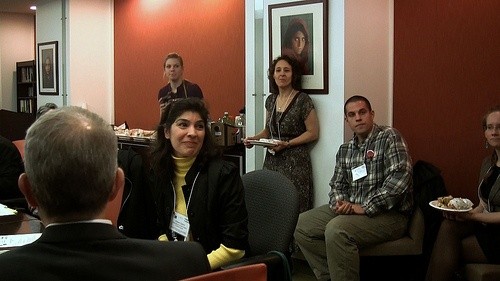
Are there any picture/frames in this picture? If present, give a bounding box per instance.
[38,41,59,95]
[268,0,329,95]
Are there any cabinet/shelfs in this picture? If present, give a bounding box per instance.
[16,60,37,115]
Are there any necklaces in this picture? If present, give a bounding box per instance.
[278,89,295,109]
[268,87,295,155]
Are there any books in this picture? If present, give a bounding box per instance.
[28,87,33,97]
[22,67,33,82]
[20,100,33,113]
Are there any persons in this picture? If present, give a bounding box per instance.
[0,103,58,219]
[149,97,249,270]
[158,53,204,120]
[0,106,211,281]
[425,108,500,281]
[243,56,318,213]
[293,95,414,281]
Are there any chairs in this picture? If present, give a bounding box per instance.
[220,169,301,281]
[359,160,446,281]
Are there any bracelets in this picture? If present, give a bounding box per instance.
[287,140,290,148]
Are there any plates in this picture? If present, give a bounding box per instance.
[247,140,277,147]
[429,200,472,212]
[0,233,42,249]
